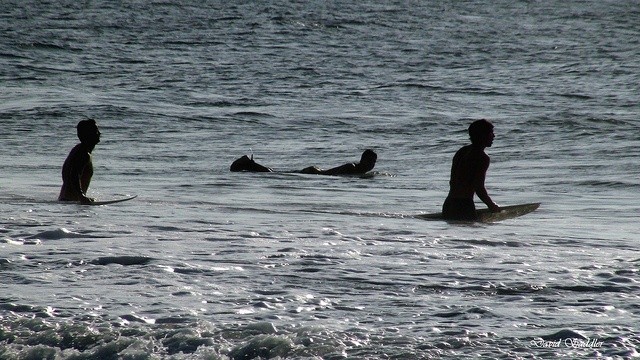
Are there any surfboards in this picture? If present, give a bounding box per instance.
[0,193,138,206]
[414,202,541,223]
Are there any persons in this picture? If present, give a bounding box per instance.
[58,119,101,204]
[442,119,500,221]
[230,150,377,176]
